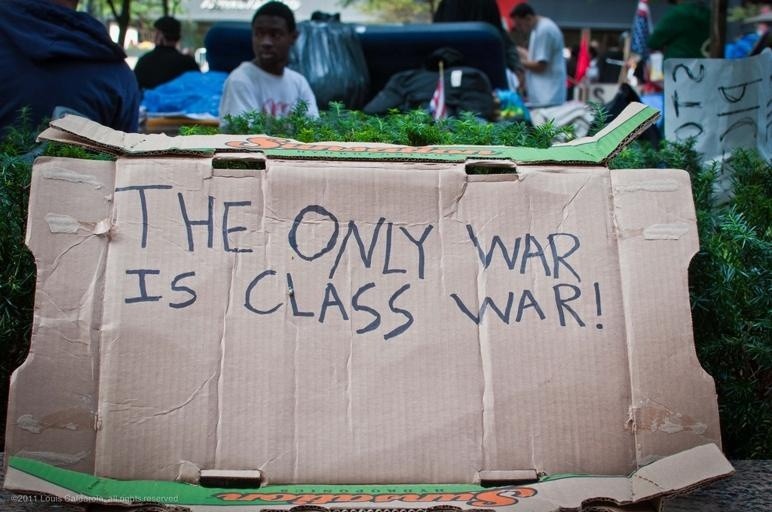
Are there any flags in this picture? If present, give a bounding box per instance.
[629,0,651,57]
[426,76,449,126]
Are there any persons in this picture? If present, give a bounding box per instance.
[0,0,142,146]
[216,1,321,130]
[432,0,528,105]
[508,1,568,111]
[133,16,201,93]
